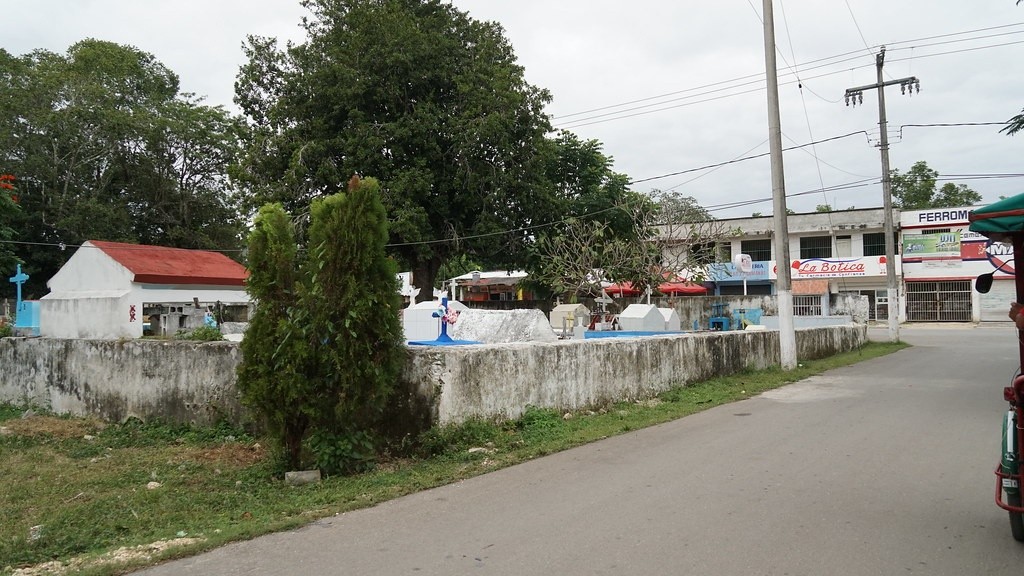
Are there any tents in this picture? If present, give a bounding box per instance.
[606,265,707,296]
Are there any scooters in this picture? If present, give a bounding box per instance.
[970,194,1024,544]
